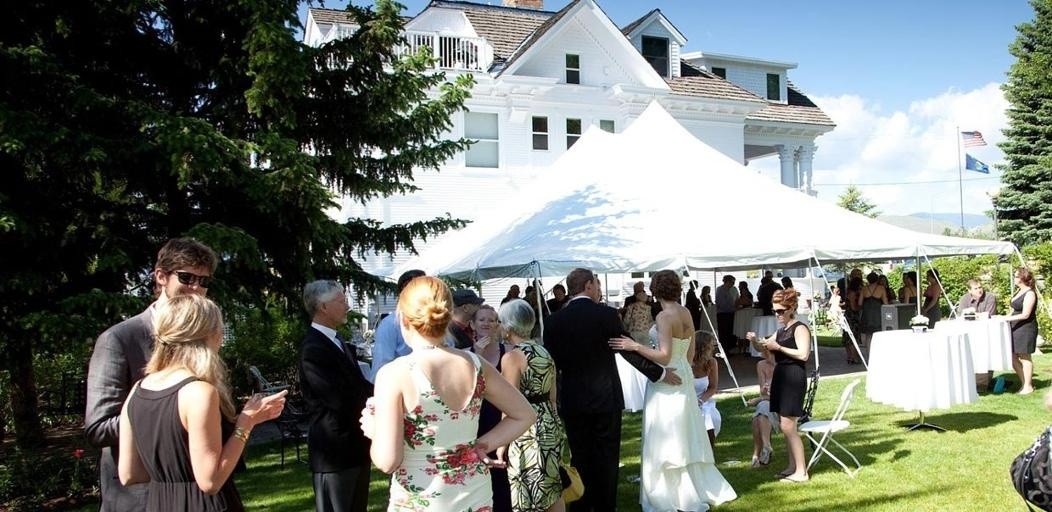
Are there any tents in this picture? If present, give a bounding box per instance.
[379,99,1023,280]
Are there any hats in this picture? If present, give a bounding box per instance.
[452,289,485,307]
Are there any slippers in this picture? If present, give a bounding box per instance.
[773,471,795,477]
[778,475,810,484]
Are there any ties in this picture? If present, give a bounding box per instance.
[335,334,357,366]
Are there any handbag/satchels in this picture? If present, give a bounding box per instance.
[559,459,585,504]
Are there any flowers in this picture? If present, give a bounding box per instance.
[909,314,930,326]
[963,307,976,315]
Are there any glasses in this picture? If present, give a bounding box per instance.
[972,286,982,290]
[770,307,790,315]
[1013,275,1020,279]
[173,270,212,289]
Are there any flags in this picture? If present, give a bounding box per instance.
[966,152,988,175]
[961,131,987,147]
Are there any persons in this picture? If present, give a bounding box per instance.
[736,281,753,306]
[456,307,518,512]
[690,330,720,450]
[372,271,454,392]
[757,271,776,302]
[522,287,535,303]
[85,237,232,512]
[545,285,570,312]
[859,273,889,362]
[850,268,864,286]
[781,276,795,290]
[360,276,537,512]
[922,269,941,329]
[699,285,713,306]
[958,279,997,317]
[1002,266,1038,396]
[546,268,683,512]
[878,274,896,302]
[622,281,655,316]
[299,281,375,511]
[607,270,738,511]
[842,278,861,366]
[117,295,287,511]
[746,336,790,467]
[623,291,654,347]
[530,280,546,337]
[446,289,484,353]
[500,285,522,305]
[717,275,738,359]
[746,289,811,481]
[759,277,783,316]
[898,273,910,300]
[686,280,701,332]
[495,300,571,512]
[904,271,920,303]
[830,288,845,329]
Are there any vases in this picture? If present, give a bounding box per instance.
[912,326,928,333]
[965,315,975,320]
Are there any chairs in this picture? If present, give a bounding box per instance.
[766,366,820,464]
[274,405,307,468]
[798,379,863,479]
[249,365,293,400]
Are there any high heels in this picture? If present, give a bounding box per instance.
[847,357,861,365]
[759,443,774,465]
[751,454,762,469]
